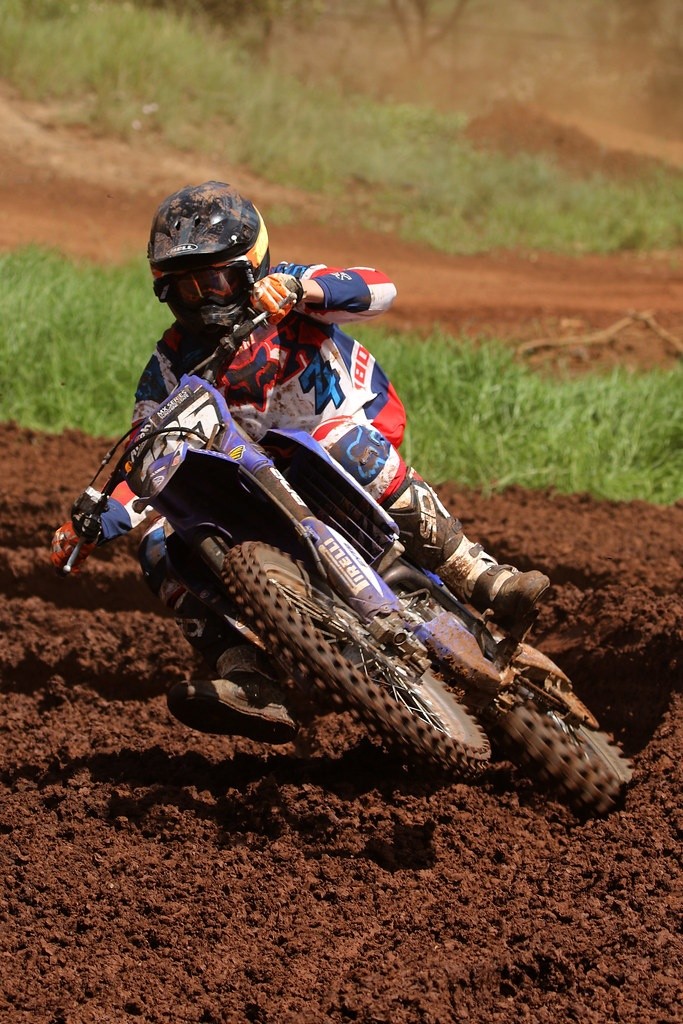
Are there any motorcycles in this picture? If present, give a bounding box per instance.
[62,281,640,816]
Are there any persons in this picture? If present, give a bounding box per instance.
[47,181,552,743]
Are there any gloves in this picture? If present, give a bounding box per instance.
[51,521,99,571]
[249,273,303,324]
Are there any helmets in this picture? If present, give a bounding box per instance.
[141,181,270,325]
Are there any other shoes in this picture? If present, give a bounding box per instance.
[441,546,550,627]
[166,648,299,745]
